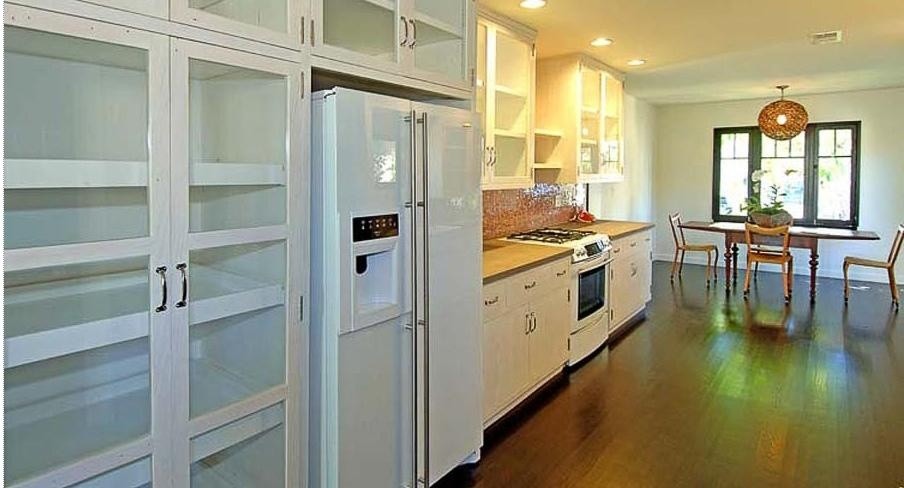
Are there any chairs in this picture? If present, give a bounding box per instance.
[843,223,904,309]
[668,208,719,291]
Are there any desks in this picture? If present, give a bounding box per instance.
[677,220,881,305]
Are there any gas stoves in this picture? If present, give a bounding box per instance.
[507,225,603,246]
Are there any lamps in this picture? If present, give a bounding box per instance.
[758,85,809,141]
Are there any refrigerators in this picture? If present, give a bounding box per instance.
[311,83,485,487]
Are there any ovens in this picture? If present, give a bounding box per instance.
[568,234,614,335]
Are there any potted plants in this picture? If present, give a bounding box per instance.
[740,168,799,228]
